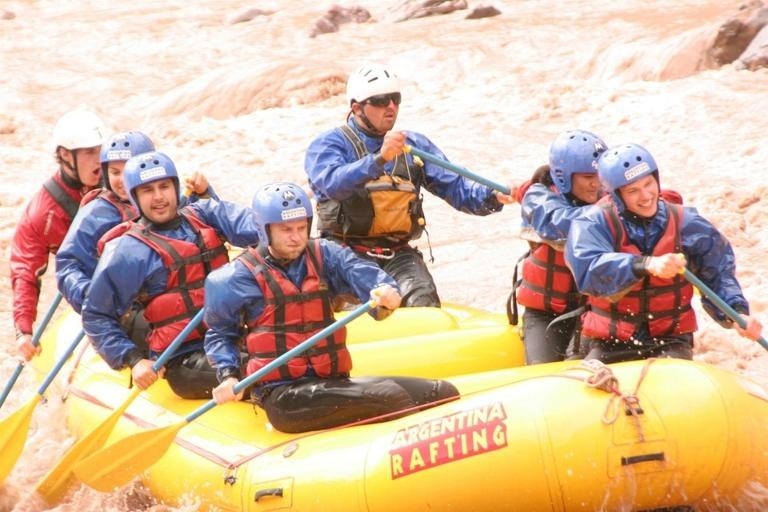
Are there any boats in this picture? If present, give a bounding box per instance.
[33,296,767,512]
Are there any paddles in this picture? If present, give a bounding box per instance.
[36,304,207,506]
[0,327,84,488]
[72,291,381,493]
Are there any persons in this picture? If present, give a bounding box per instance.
[203,180,461,435]
[80,150,261,400]
[55,130,212,367]
[6,108,107,360]
[562,143,764,364]
[302,61,515,308]
[513,131,682,364]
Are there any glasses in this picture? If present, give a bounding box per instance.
[362,93,401,108]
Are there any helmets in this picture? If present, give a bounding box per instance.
[51,108,180,217]
[252,183,312,247]
[549,130,661,214]
[347,62,402,109]
